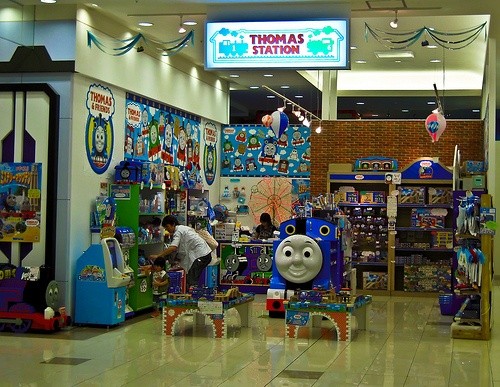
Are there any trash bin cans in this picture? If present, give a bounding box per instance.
[464,295,481,319]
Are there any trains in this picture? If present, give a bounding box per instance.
[265,217,344,318]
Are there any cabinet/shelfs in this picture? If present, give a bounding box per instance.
[109,158,491,341]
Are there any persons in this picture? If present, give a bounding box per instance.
[149,257,171,318]
[149,215,212,287]
[251,213,277,240]
